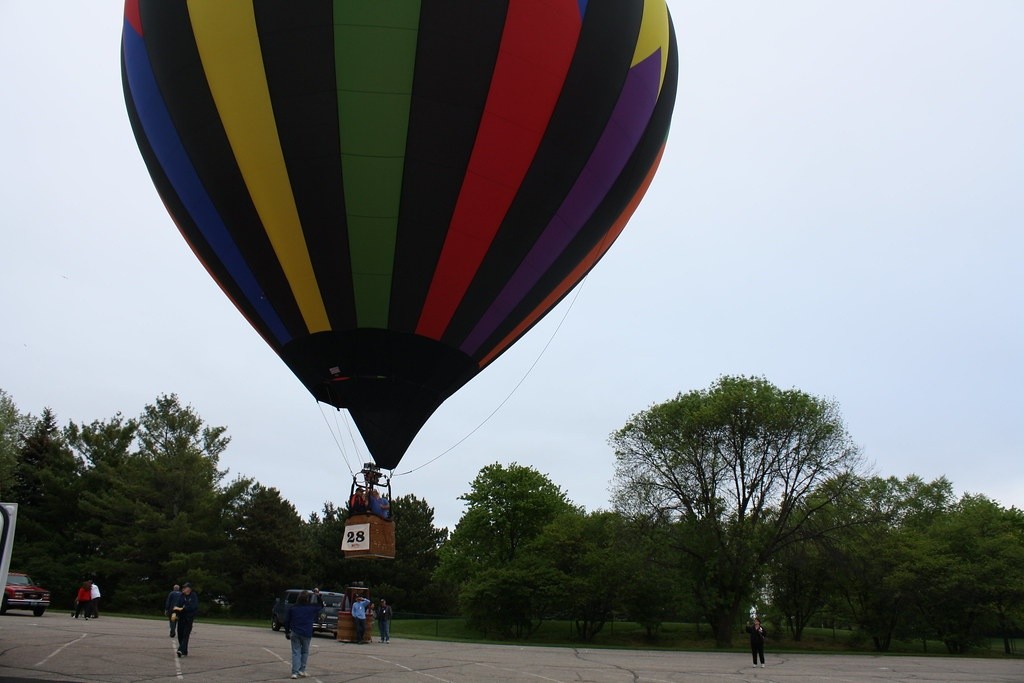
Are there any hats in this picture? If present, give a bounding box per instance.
[182,582,191,587]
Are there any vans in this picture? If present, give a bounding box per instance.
[270,589,350,641]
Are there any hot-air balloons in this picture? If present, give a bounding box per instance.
[127,0,682,560]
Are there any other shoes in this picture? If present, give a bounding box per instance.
[298,671,309,677]
[387,640,389,644]
[181,655,187,658]
[291,674,297,679]
[378,640,383,643]
[177,651,182,657]
[753,664,757,667]
[761,664,764,667]
[358,642,362,643]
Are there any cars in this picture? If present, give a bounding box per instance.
[0,572,52,616]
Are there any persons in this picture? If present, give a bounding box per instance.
[350,486,390,519]
[352,596,370,644]
[165,585,183,638]
[171,583,199,657]
[286,586,324,678]
[366,598,393,643]
[73,579,101,619]
[746,619,767,668]
